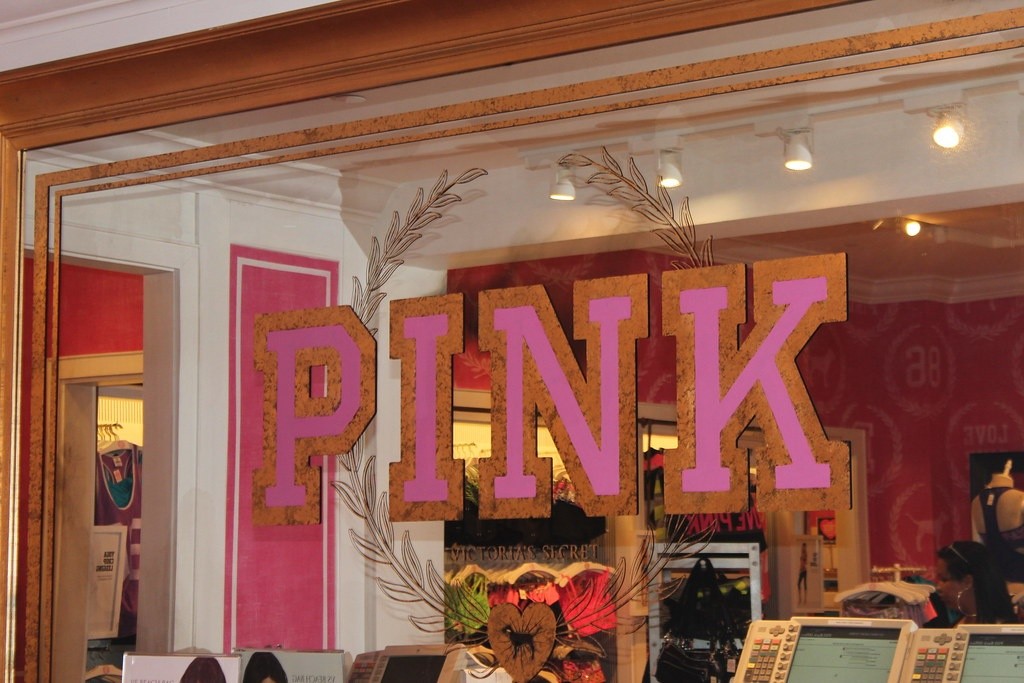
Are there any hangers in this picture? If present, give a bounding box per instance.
[96,423,143,455]
[832,563,936,608]
[441,541,617,591]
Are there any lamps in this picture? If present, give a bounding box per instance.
[549,162,576,202]
[929,104,966,148]
[656,149,684,189]
[775,124,815,171]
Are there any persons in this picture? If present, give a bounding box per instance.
[971,460,1024,598]
[242,652,288,683]
[180,658,226,683]
[936,541,1015,623]
